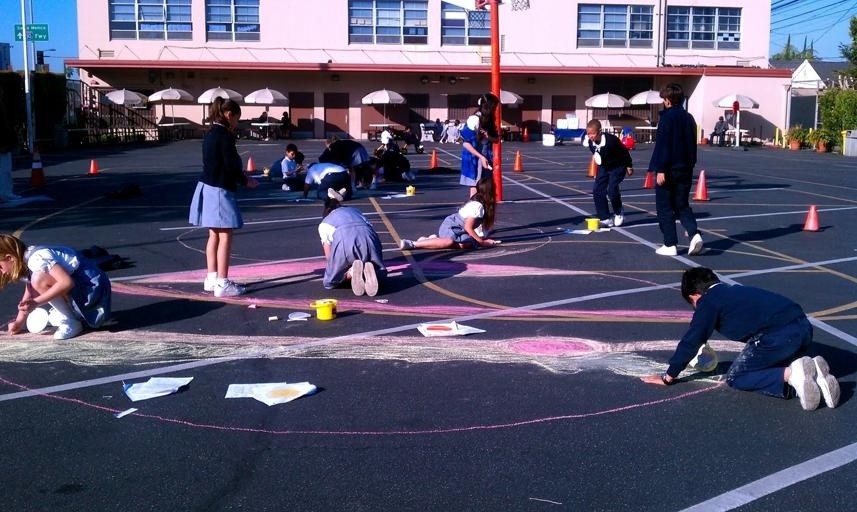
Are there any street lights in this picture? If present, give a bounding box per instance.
[41,49,56,64]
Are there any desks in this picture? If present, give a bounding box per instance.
[634,125,658,145]
[250,122,284,142]
[600,126,623,139]
[368,123,400,133]
[708,127,751,148]
[155,122,192,141]
[553,126,587,146]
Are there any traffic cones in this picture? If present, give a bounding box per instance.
[583,154,597,179]
[522,126,529,142]
[245,153,255,172]
[30,150,44,182]
[430,149,437,168]
[87,159,101,176]
[691,169,710,200]
[801,204,820,232]
[642,171,655,189]
[513,149,524,172]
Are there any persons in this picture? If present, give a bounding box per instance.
[317,198,387,297]
[709,116,728,147]
[259,111,462,203]
[399,178,497,250]
[0,233,111,341]
[640,266,842,412]
[187,96,259,297]
[586,118,634,228]
[459,94,499,197]
[647,82,703,256]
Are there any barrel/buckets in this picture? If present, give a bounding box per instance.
[310,297,338,321]
[585,218,600,231]
[406,184,415,196]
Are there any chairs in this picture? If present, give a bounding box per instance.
[418,123,435,143]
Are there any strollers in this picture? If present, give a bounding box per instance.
[388,126,425,154]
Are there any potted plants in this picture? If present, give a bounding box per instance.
[807,126,834,154]
[782,122,807,150]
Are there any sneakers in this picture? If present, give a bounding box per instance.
[398,238,417,251]
[202,278,214,292]
[787,354,822,413]
[213,282,247,297]
[53,317,84,341]
[350,258,365,297]
[809,354,842,409]
[326,187,345,204]
[613,210,625,227]
[687,233,704,256]
[656,243,678,256]
[599,218,613,227]
[362,260,380,298]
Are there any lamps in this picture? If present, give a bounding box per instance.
[448,76,457,89]
[419,75,430,85]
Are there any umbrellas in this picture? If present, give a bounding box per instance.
[102,88,148,128]
[584,92,630,127]
[629,89,664,141]
[244,87,289,137]
[360,88,407,131]
[713,93,759,143]
[478,89,524,126]
[198,86,243,105]
[147,86,194,123]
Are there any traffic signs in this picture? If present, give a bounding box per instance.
[14,24,48,41]
